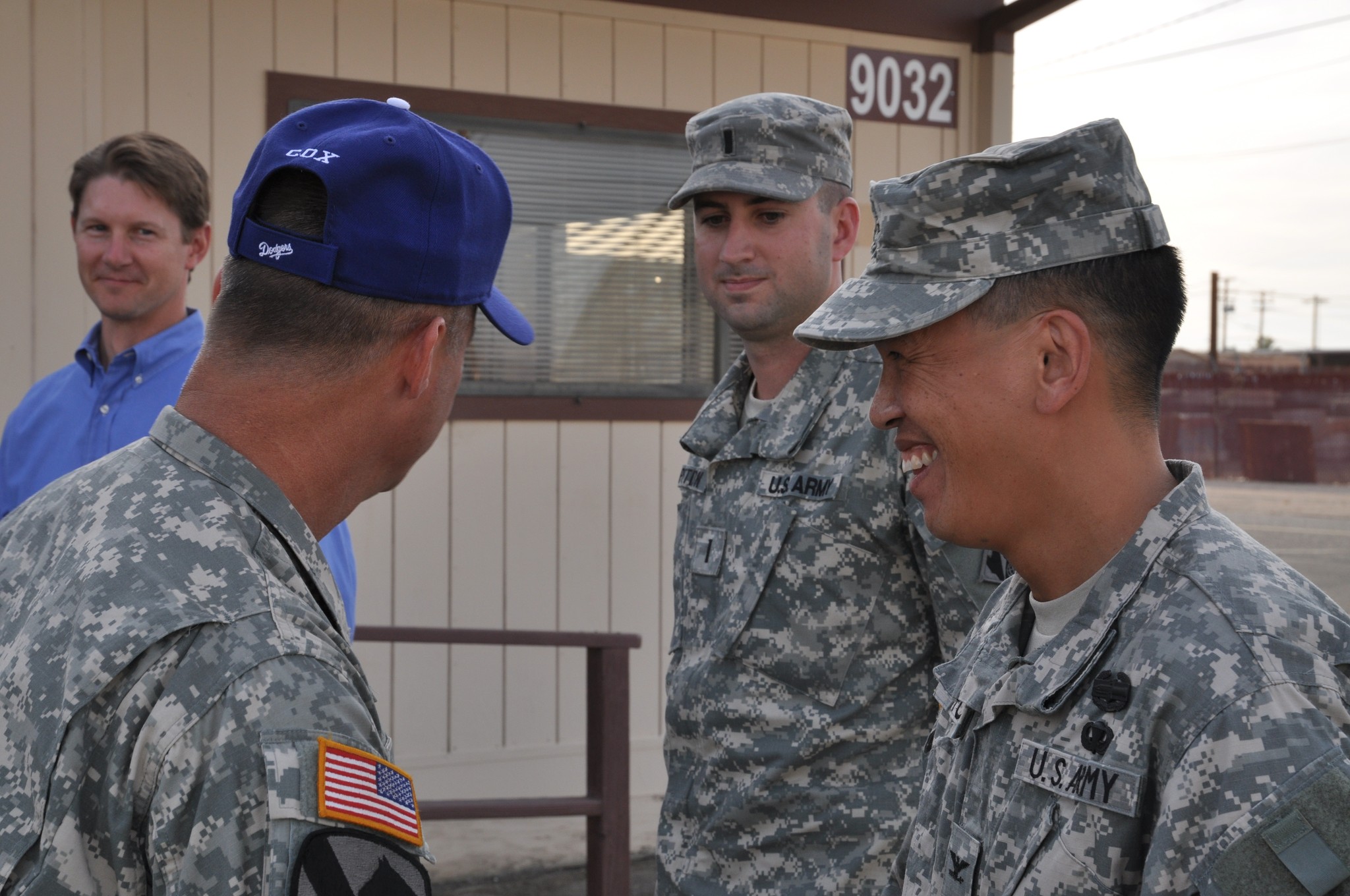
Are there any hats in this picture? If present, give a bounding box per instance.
[668,92,853,210]
[226,98,536,347]
[793,118,1170,352]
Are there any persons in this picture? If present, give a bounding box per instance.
[0,132,357,643]
[0,96,533,896]
[795,119,1349,895]
[658,92,1014,895]
